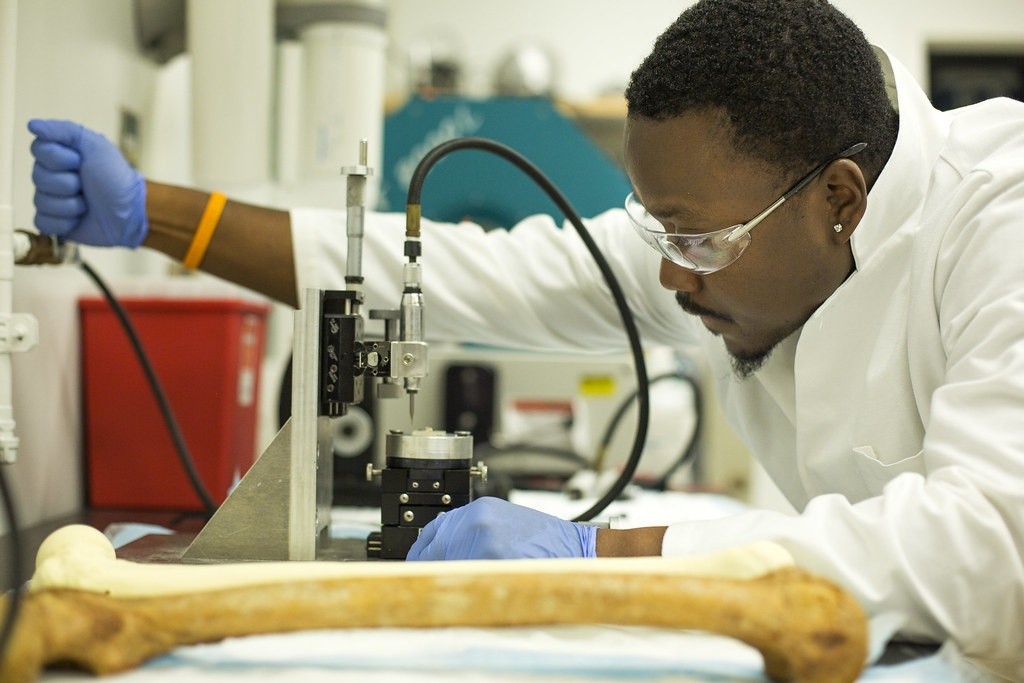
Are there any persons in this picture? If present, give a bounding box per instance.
[26,2,1024,683]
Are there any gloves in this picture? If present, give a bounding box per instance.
[404,495,600,562]
[28,120,148,249]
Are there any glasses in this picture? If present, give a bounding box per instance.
[624,142,869,276]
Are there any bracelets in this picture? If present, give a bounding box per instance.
[181,193,226,268]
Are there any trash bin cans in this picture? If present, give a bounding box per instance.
[78,298,275,512]
